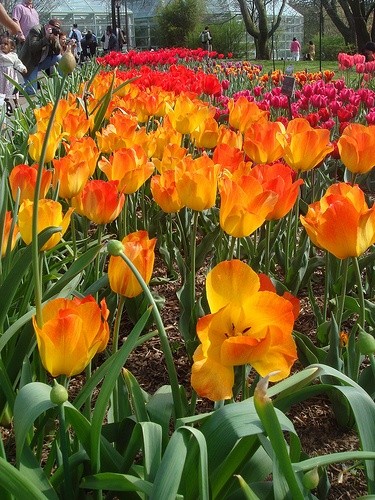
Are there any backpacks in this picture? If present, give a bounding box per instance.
[70,29,79,43]
[202,31,208,43]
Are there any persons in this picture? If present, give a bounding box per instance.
[308,41,315,61]
[290,37,301,60]
[199,26,212,50]
[0,0,126,106]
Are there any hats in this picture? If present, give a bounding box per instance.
[49,19,61,29]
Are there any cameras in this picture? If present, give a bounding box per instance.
[51,28,60,36]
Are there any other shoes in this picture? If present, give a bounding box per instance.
[7,107,12,116]
